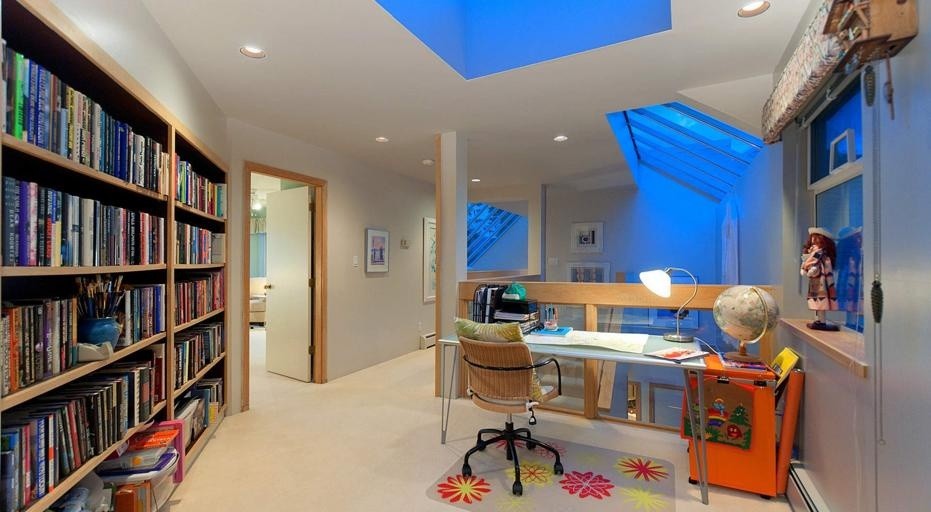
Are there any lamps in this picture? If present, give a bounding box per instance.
[638,265,699,343]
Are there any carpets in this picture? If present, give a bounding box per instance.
[426,429,676,512]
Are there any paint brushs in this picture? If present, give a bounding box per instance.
[70,274,126,318]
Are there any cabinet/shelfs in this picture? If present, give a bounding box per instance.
[0,2,231,512]
[682,351,777,500]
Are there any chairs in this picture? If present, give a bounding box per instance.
[457,333,563,496]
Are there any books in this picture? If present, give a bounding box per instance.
[476,287,541,334]
[531,326,573,336]
[644,346,710,362]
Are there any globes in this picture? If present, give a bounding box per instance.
[713,285,780,362]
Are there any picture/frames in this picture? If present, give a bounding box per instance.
[364,228,389,273]
[566,221,611,282]
[424,216,436,302]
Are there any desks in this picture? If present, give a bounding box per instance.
[436,327,711,505]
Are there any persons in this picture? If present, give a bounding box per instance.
[838,224,865,332]
[799,226,838,328]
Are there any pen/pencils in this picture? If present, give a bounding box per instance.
[545,303,558,322]
